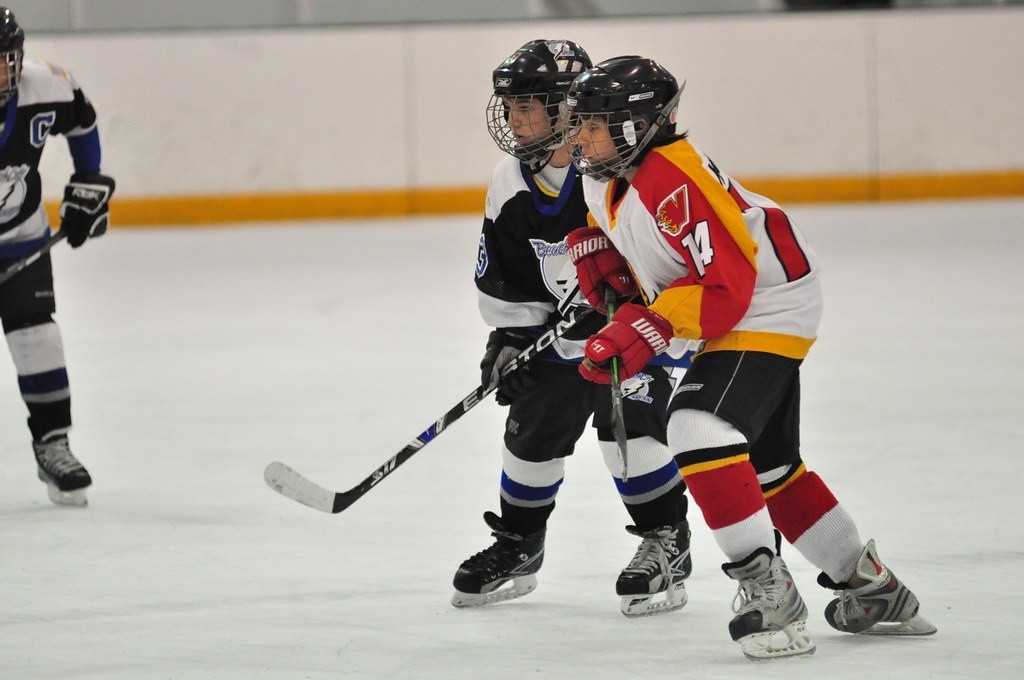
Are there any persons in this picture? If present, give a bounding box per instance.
[561,54,936,657]
[450,38,692,618]
[0,11,116,506]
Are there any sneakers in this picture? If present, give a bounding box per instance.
[450,500,556,609]
[817,538,938,635]
[721,529,816,658]
[28,413,92,505]
[615,494,693,616]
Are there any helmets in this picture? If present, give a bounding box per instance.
[492,40,593,162]
[567,55,679,184]
[0,7,24,99]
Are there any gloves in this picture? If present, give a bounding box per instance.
[60,172,115,248]
[481,325,545,406]
[578,301,675,385]
[568,227,639,315]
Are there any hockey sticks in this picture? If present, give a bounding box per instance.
[262,298,598,516]
[601,285,631,484]
[1,227,64,282]
